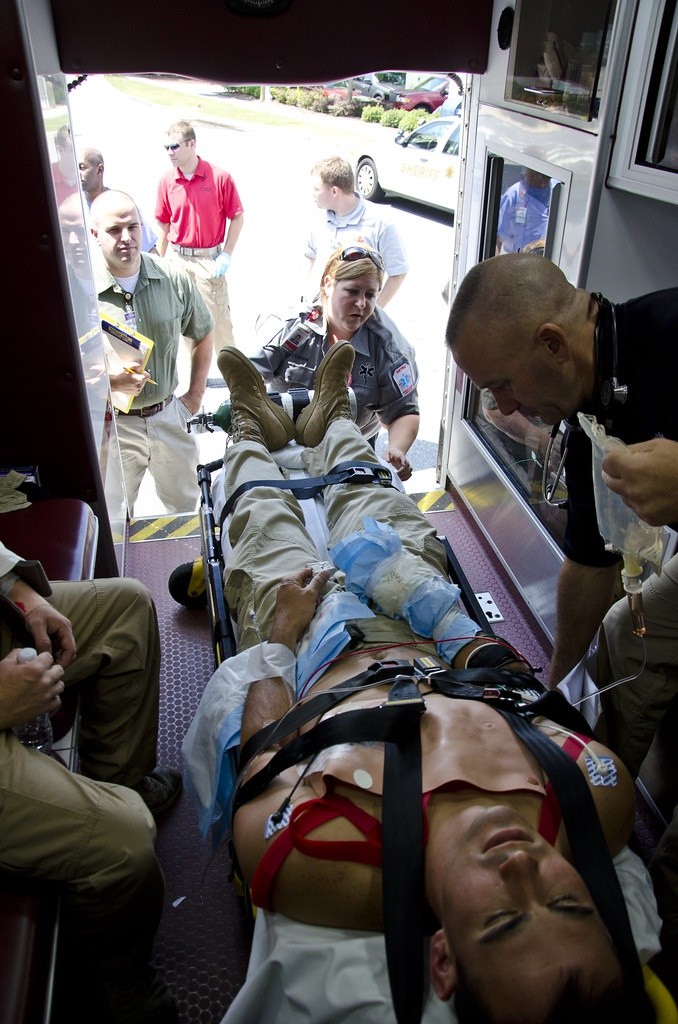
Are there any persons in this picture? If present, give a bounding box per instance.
[494,148,560,254]
[154,121,242,352]
[248,241,419,480]
[220,339,637,1024]
[296,154,409,310]
[1,543,181,1023]
[91,191,216,515]
[79,146,160,256]
[445,252,677,774]
[52,128,107,436]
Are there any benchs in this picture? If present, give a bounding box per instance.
[0,498,101,1024]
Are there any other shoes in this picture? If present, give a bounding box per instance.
[85,976,177,1024]
[294,340,356,448]
[218,347,297,456]
[127,764,182,825]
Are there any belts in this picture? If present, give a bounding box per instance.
[169,241,221,257]
[118,394,175,417]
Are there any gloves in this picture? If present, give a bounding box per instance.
[209,252,231,279]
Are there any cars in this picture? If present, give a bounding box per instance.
[329,75,395,101]
[350,114,521,218]
[384,75,453,114]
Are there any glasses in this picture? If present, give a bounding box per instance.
[163,139,192,151]
[339,247,384,269]
